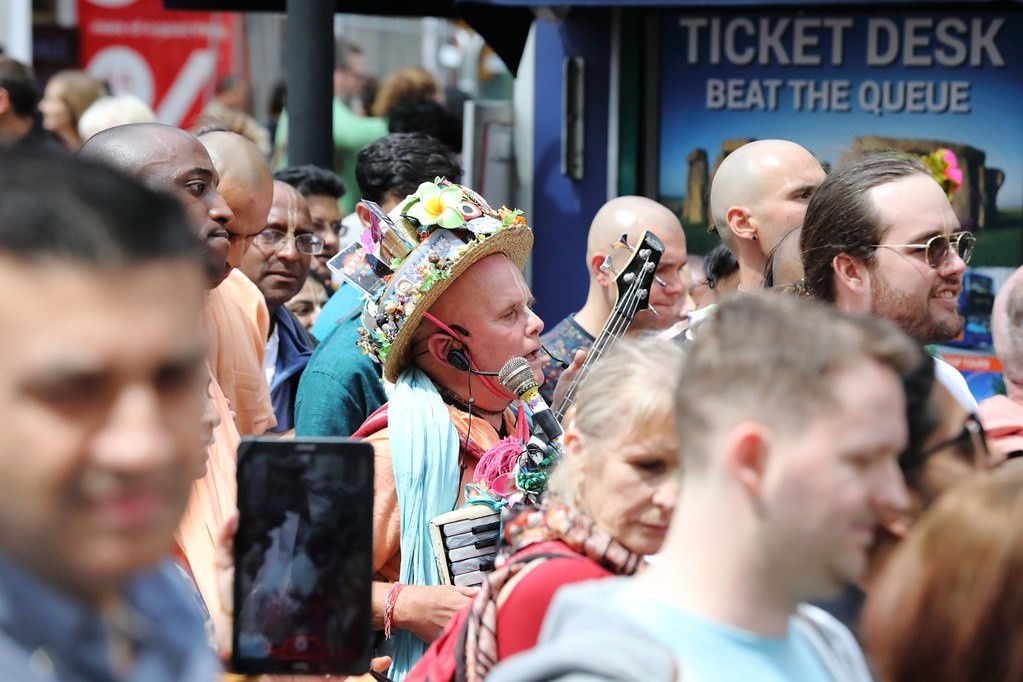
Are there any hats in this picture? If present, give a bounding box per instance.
[354,175,535,384]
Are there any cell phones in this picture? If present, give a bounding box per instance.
[230,437,375,677]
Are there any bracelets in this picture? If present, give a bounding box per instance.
[383,582,405,641]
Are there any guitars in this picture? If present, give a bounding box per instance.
[552,230,669,438]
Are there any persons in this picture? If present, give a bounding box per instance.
[332,565,371,658]
[299,455,368,528]
[0,40,1023,682]
[239,582,296,647]
[285,531,340,627]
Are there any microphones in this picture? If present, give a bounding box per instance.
[499,357,562,441]
[541,346,570,369]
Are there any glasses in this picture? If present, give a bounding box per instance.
[866,230,977,270]
[314,222,348,237]
[256,228,323,255]
